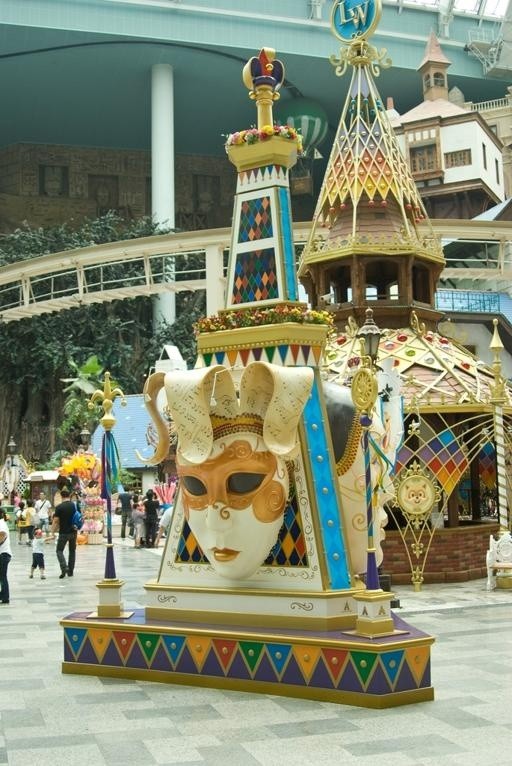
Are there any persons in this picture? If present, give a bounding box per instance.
[117,486,160,549]
[0,499,10,521]
[51,490,77,578]
[30,528,54,579]
[153,506,174,548]
[0,508,12,603]
[13,491,52,546]
[133,360,314,581]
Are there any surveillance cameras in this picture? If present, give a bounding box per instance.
[320,294,333,304]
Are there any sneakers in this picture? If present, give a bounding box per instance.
[60,567,70,578]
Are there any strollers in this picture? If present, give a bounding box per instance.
[139,513,161,548]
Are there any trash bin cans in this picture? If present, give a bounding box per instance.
[1,505,16,531]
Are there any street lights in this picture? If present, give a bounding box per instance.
[80,422,91,452]
[8,435,19,506]
[356,307,399,610]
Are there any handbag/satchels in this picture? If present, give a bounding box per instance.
[72,511,84,531]
